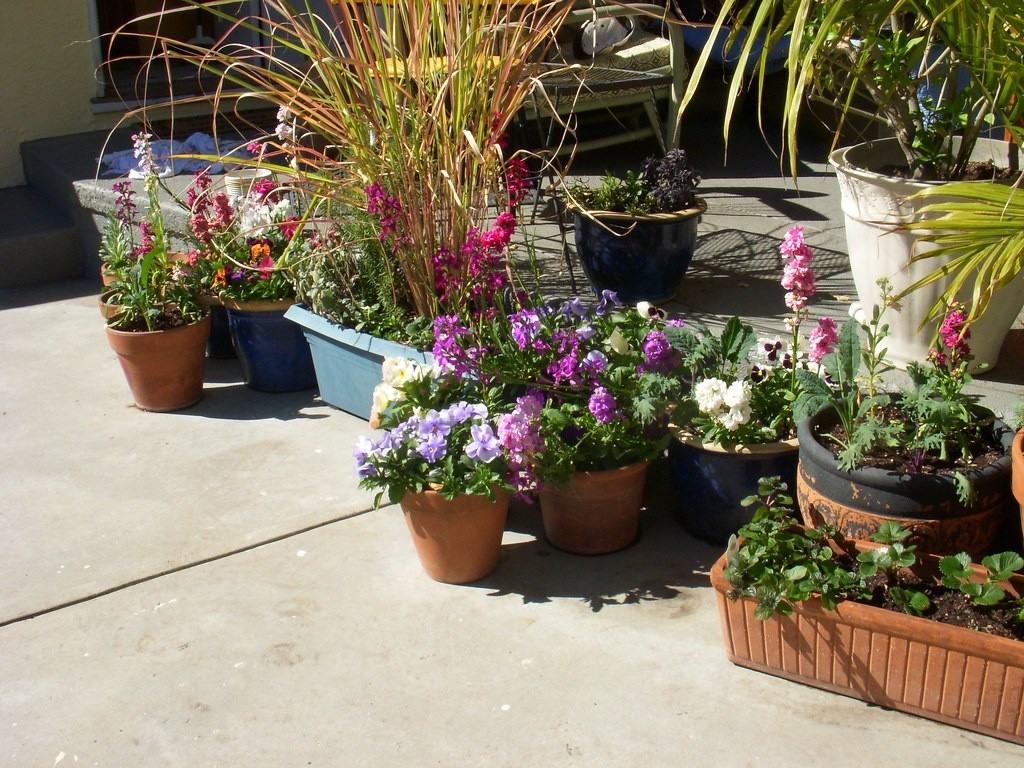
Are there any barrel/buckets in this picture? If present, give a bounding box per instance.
[225,166,273,203]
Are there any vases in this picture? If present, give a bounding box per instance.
[283,301,480,433]
[205,296,238,359]
[106,304,211,412]
[220,297,318,392]
[668,400,799,546]
[537,428,652,554]
[99,283,188,319]
[400,471,513,584]
[796,392,1016,565]
[101,252,189,290]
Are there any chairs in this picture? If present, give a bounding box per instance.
[509,2,693,155]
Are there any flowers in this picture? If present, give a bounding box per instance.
[96,103,1004,511]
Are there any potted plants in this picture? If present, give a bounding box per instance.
[1013,401,1024,537]
[710,476,1024,747]
[780,0,1024,374]
[565,146,708,307]
